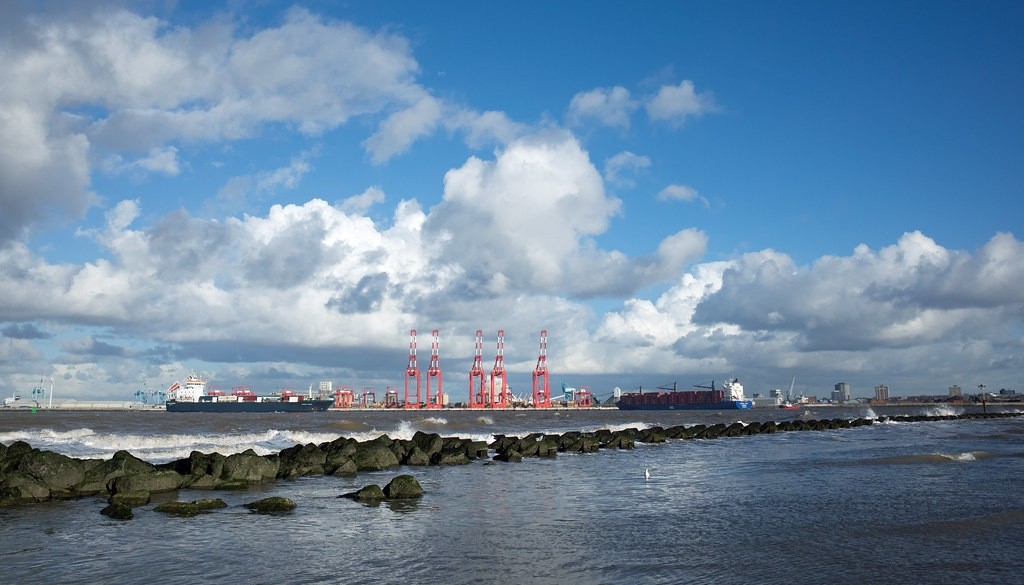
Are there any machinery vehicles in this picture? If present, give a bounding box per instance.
[787,376,797,403]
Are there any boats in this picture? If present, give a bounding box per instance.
[779,400,793,408]
[613,382,752,410]
[166,376,336,413]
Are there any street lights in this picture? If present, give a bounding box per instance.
[978,384,987,413]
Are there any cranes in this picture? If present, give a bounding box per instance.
[655,381,677,392]
[693,380,715,391]
